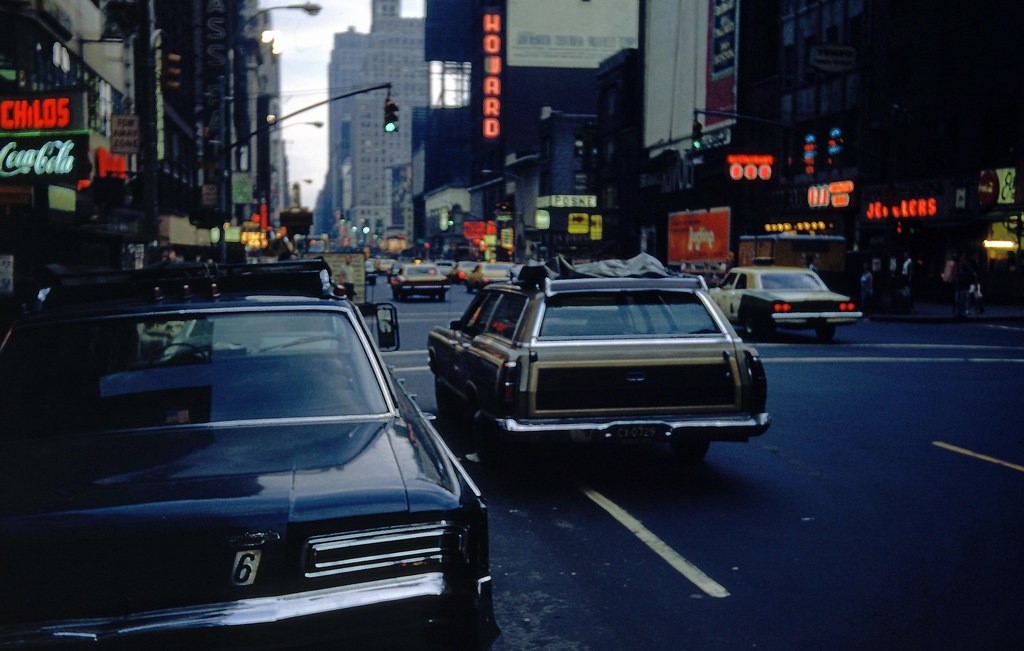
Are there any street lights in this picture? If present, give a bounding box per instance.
[219,4,323,272]
[255,121,323,247]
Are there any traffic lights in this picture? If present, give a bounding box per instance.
[691,122,703,153]
[384,98,399,132]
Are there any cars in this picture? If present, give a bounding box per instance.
[428,272,771,474]
[708,266,863,342]
[0,283,503,651]
[450,261,511,293]
[390,264,450,302]
